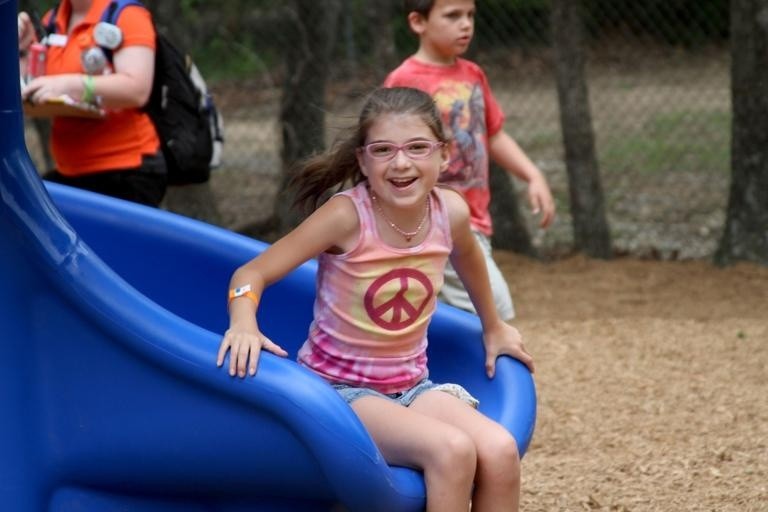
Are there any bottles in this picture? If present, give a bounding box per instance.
[79,37,111,76]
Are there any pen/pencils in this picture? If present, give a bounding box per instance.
[20,78,32,104]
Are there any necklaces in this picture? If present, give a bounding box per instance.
[371,186,429,242]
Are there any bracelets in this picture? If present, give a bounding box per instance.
[80,73,97,104]
[227,285,260,306]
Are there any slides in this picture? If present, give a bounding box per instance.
[0,0,537,511]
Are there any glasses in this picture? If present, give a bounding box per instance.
[361,139,444,161]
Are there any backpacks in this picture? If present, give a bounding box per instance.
[99,0,225,188]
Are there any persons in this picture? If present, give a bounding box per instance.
[381,1,559,321]
[215,87,536,511]
[17,0,168,209]
